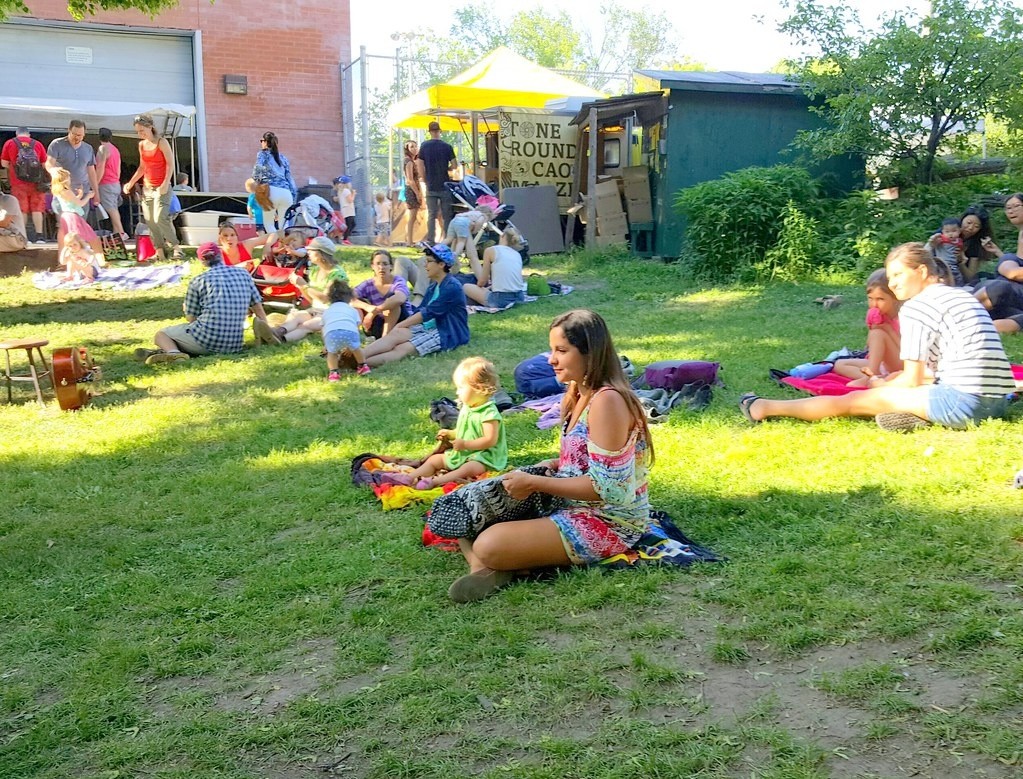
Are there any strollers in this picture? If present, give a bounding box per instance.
[250,202,332,309]
[443,174,530,265]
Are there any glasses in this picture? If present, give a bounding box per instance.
[424,258,439,264]
[260,140,267,143]
[134,117,149,122]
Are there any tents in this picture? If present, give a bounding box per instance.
[388,47,612,247]
[0,96,200,189]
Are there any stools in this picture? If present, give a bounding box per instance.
[629,222,653,252]
[0,339,54,408]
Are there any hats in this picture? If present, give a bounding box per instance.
[333,176,352,183]
[428,121,442,132]
[305,236,337,256]
[419,240,455,266]
[196,241,222,263]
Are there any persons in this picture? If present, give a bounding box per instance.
[739,193,1023,431]
[381,358,508,491]
[0,116,526,382]
[427,308,656,600]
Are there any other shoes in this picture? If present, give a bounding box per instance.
[381,472,414,487]
[342,239,352,245]
[415,478,434,490]
[253,316,282,346]
[132,348,191,370]
[146,251,186,262]
[449,567,512,603]
[356,364,371,375]
[328,370,341,382]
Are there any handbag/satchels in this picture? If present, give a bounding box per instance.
[429,396,460,429]
[527,272,550,296]
[100,232,128,261]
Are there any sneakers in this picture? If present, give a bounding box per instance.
[122,232,130,241]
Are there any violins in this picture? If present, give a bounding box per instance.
[51,346,101,410]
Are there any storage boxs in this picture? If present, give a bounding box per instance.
[567,164,654,249]
[180,211,257,245]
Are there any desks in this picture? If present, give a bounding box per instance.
[172,190,251,210]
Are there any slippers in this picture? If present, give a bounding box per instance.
[875,411,933,432]
[738,393,764,423]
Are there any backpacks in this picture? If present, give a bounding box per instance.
[12,137,45,183]
[644,359,718,390]
